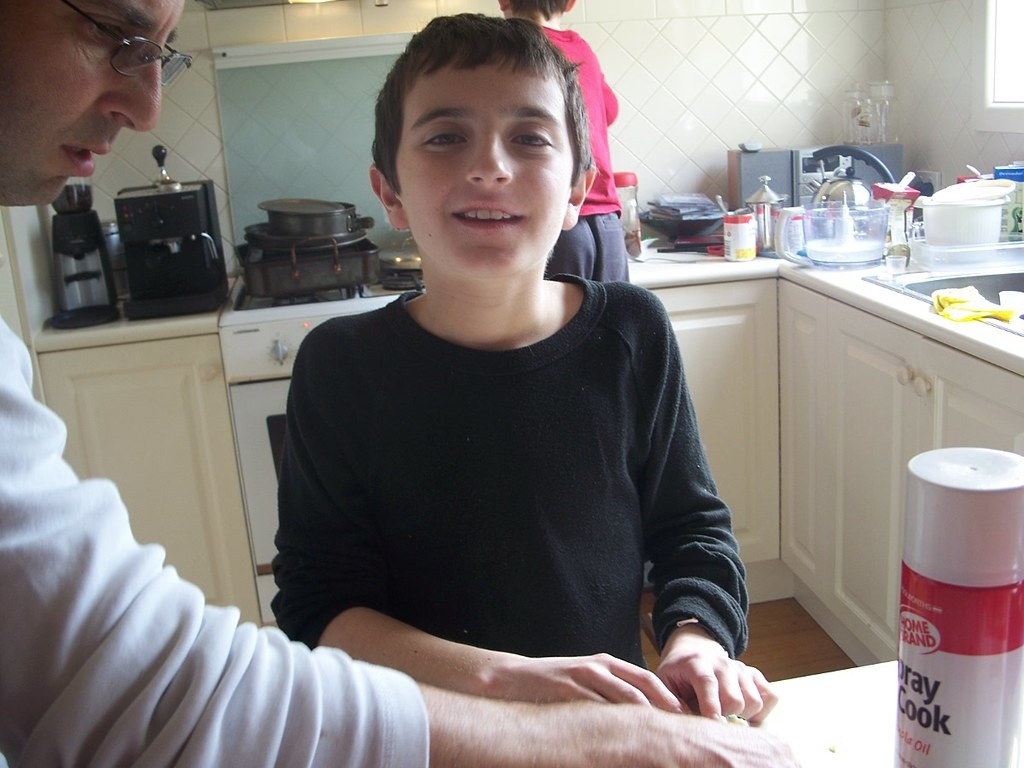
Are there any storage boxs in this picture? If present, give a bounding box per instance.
[993,166,1024,241]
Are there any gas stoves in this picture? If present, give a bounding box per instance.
[221,258,427,324]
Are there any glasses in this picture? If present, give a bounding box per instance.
[61,0,192,86]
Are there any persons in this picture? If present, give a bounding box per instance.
[498,0,629,283]
[0,0,805,768]
[270,13,781,727]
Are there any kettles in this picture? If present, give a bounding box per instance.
[805,145,898,207]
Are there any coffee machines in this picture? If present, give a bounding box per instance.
[114,148,230,321]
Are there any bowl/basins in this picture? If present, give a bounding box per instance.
[917,194,1010,249]
[803,205,888,267]
[638,203,730,239]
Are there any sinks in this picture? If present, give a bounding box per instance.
[860,266,1024,339]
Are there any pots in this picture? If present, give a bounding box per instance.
[234,202,384,298]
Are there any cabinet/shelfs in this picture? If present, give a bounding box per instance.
[35,278,263,629]
[778,277,1024,663]
[648,278,782,562]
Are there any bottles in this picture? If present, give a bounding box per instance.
[843,74,902,148]
[611,173,641,260]
[722,211,757,262]
[745,175,787,251]
[771,208,803,255]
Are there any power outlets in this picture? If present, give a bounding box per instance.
[917,171,941,194]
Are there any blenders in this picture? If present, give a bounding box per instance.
[50,171,120,330]
